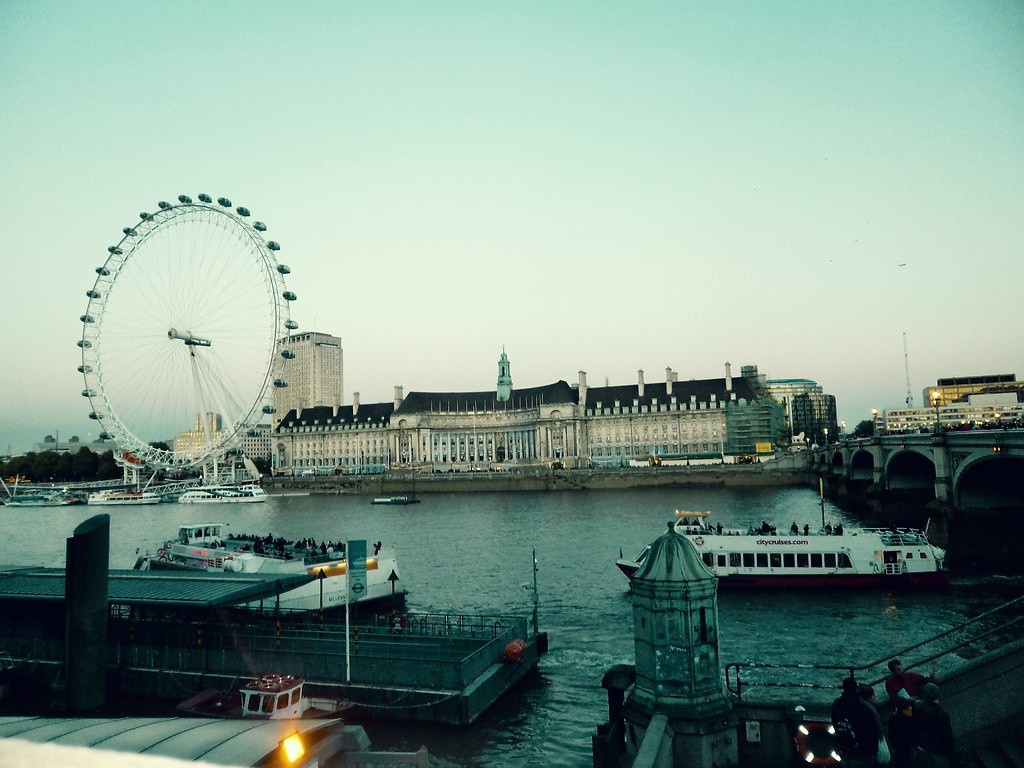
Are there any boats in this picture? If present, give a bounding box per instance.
[5,491,79,505]
[130,522,411,619]
[88,491,161,505]
[178,484,269,502]
[370,496,422,505]
[615,478,950,589]
[0,547,549,725]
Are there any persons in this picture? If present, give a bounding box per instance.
[826,522,844,536]
[717,521,724,535]
[205,533,345,564]
[803,523,810,535]
[749,521,777,537]
[791,521,798,535]
[373,540,382,556]
[830,660,955,768]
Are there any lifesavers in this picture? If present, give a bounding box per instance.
[694,536,705,546]
[791,722,843,764]
[244,674,301,693]
[388,614,406,632]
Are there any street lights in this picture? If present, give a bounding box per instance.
[824,428,828,445]
[931,392,941,432]
[842,420,846,434]
[872,409,878,435]
